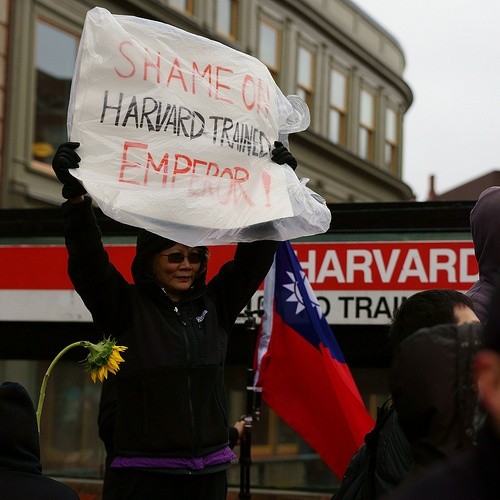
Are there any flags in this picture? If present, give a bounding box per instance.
[253,241,377,481]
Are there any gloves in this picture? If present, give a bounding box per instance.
[52,142,87,199]
[271,140,297,171]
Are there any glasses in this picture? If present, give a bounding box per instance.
[158,252,203,263]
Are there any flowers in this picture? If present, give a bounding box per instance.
[34,334,129,436]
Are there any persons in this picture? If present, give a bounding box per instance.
[52,141,298,500]
[0,382,80,500]
[331,185,500,500]
